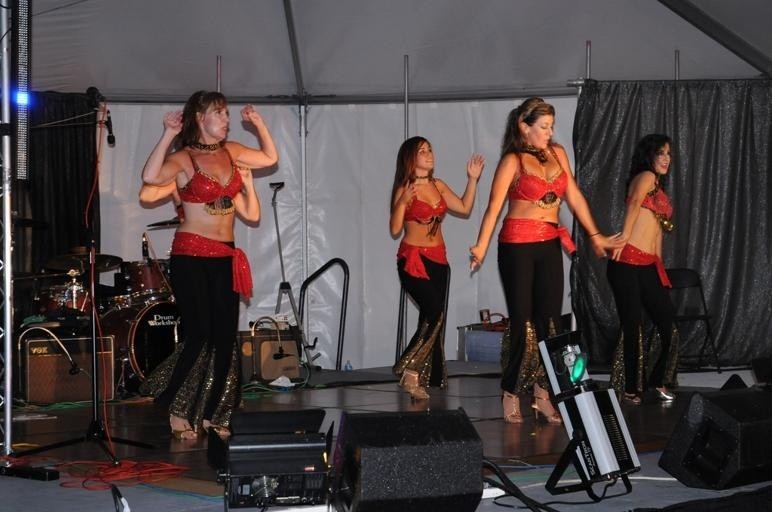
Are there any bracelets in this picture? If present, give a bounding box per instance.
[588,232,602,238]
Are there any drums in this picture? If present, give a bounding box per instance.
[100,300,184,389]
[102,294,134,312]
[119,260,171,306]
[40,284,92,315]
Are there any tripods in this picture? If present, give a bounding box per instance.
[12,243,156,467]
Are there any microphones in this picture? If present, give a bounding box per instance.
[103,109,117,148]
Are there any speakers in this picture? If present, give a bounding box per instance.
[657,387,772,489]
[20,334,117,404]
[329,406,483,512]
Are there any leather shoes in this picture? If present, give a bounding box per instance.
[655,388,677,402]
[530,396,562,425]
[621,391,643,407]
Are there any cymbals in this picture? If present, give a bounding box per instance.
[12,215,50,229]
[41,253,122,273]
[147,220,181,227]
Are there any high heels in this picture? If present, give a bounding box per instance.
[396,369,431,400]
[168,411,232,442]
[501,391,523,424]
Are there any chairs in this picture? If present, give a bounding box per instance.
[658,265,723,375]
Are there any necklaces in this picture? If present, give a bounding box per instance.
[413,174,430,180]
[195,141,223,153]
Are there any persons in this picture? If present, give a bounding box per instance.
[140,153,259,411]
[399,394,429,414]
[164,431,229,484]
[386,133,485,404]
[142,88,280,444]
[467,93,625,427]
[496,424,559,465]
[604,131,684,406]
